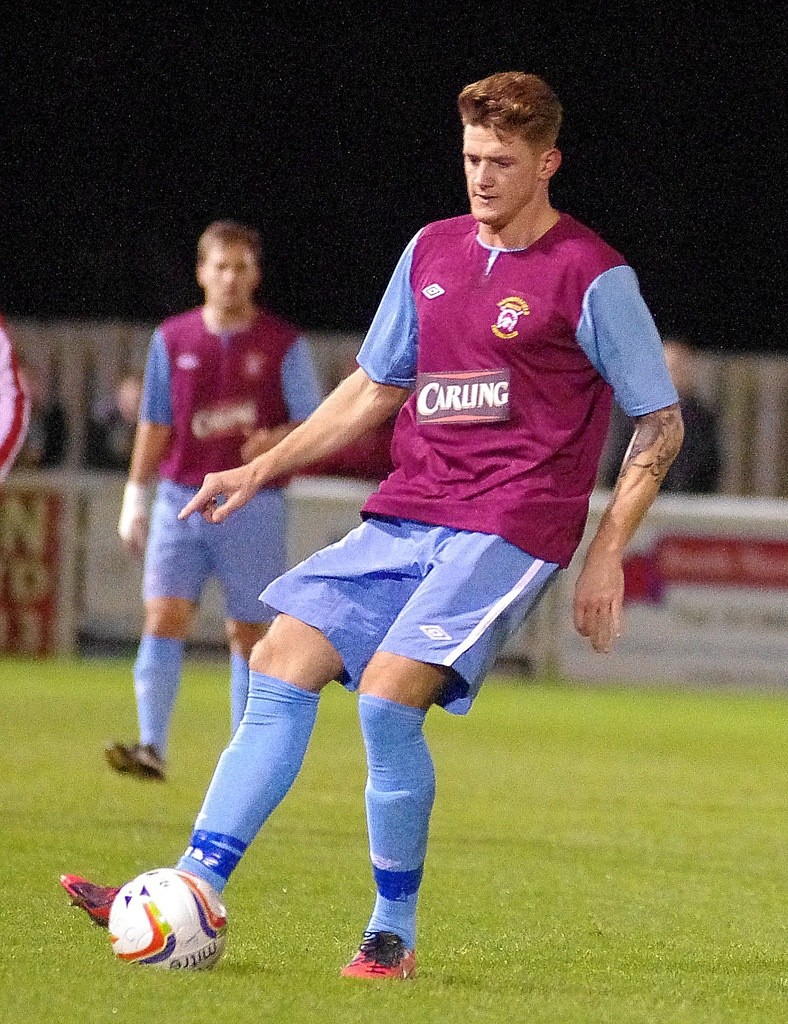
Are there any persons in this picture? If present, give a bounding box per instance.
[297,356,395,483]
[61,69,685,986]
[1,316,31,488]
[102,220,326,781]
[13,362,145,470]
[602,342,728,497]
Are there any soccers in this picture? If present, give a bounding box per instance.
[107,867,229,972]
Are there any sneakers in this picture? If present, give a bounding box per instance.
[106,742,166,783]
[337,928,418,982]
[61,874,124,928]
